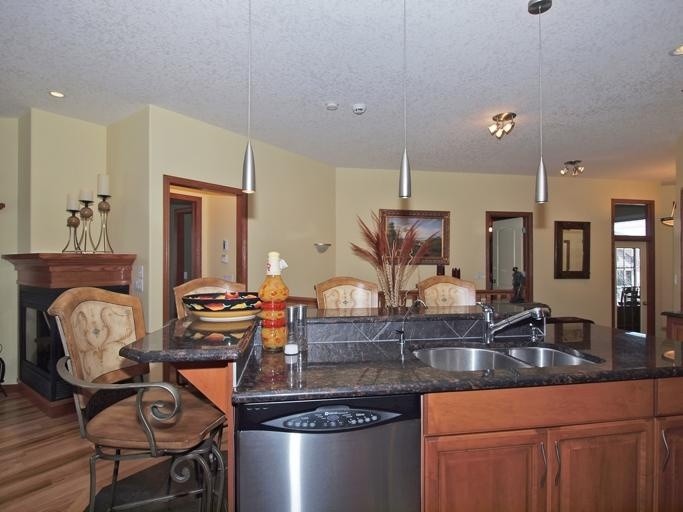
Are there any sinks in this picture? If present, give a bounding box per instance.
[504,343,606,367]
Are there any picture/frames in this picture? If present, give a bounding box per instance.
[379,209,449,264]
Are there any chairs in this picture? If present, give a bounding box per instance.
[46,287,226,512]
[416,275,476,306]
[173,277,247,319]
[314,276,379,309]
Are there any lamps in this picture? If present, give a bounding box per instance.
[315,243,331,254]
[488,112,517,138]
[242,0,254,195]
[661,201,677,226]
[560,160,585,176]
[398,0,411,198]
[528,0,552,205]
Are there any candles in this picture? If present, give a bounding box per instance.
[79,190,93,202]
[98,173,111,196]
[67,193,80,210]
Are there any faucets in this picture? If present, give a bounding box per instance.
[482,302,551,345]
[394,299,429,344]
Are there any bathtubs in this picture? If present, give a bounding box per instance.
[409,344,534,372]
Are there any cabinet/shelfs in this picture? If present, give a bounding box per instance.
[421,377,683,512]
[2,254,136,418]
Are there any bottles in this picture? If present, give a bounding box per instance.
[258,251,289,353]
[255,347,307,391]
[286,303,308,355]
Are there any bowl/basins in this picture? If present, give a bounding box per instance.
[181,292,263,322]
[182,320,254,345]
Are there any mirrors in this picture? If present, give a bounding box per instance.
[555,220,590,279]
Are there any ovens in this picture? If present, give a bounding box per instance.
[233,393,420,512]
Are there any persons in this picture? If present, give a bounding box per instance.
[511,266,523,297]
